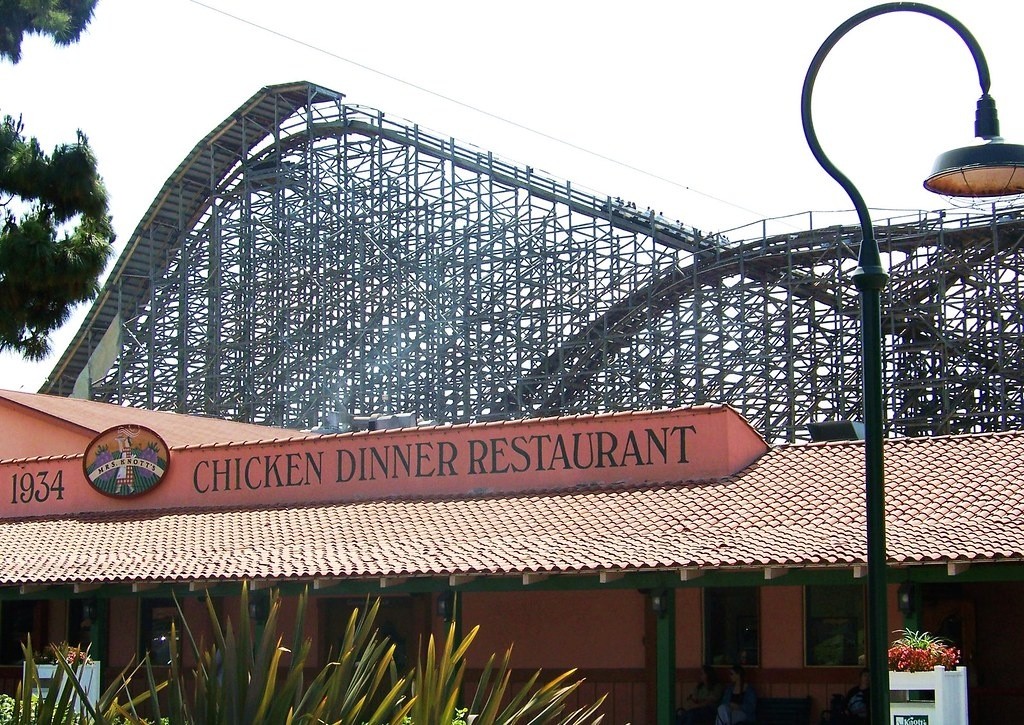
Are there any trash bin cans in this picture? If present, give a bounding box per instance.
[887,665,969,725]
[21,659,101,716]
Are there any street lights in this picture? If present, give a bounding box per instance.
[798,2,1023,724]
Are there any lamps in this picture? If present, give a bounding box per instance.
[651,596,665,612]
[898,582,915,615]
[82,601,98,619]
[249,602,263,620]
[437,595,452,617]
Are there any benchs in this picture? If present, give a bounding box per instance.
[676,694,813,725]
[819,694,871,725]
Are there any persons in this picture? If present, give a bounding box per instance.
[841,668,871,725]
[675,664,757,725]
